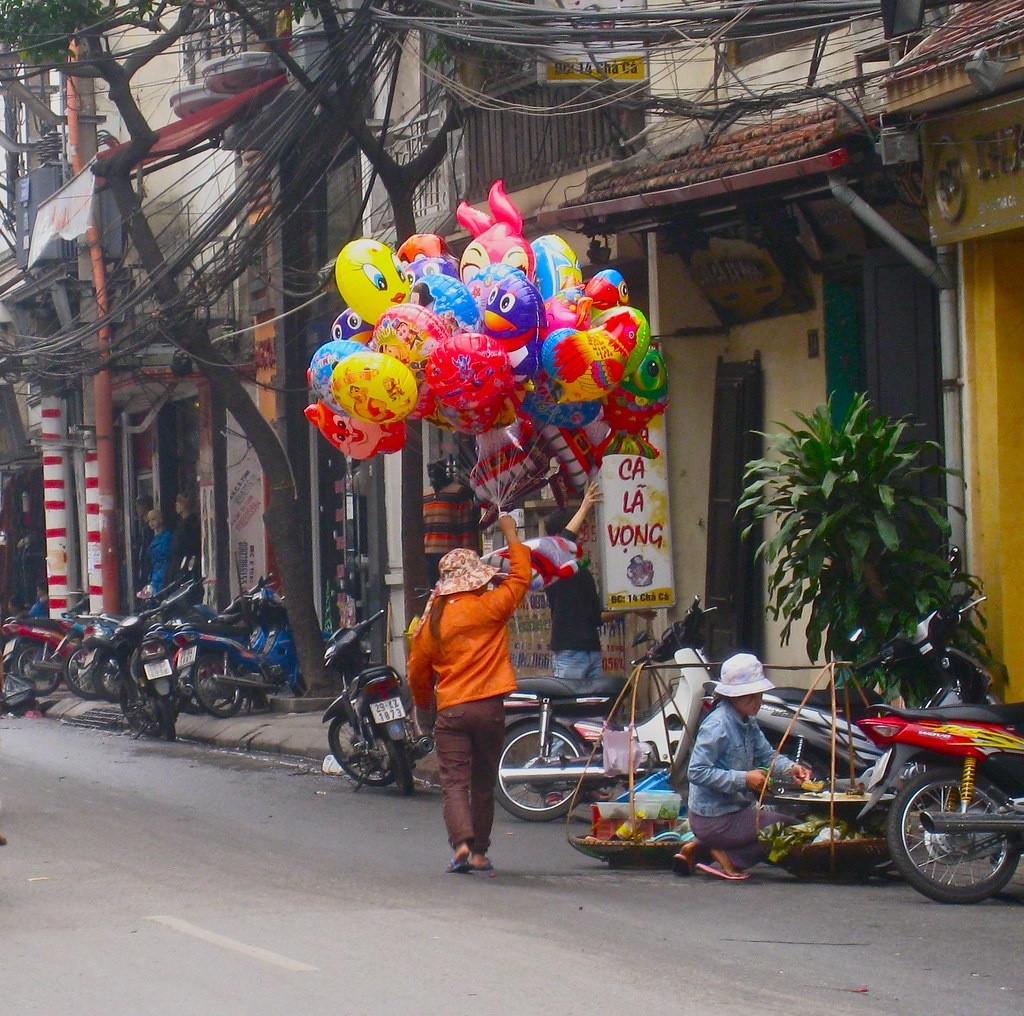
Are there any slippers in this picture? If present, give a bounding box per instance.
[447,858,491,874]
[672,853,750,880]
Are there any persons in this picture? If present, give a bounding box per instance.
[543,481,659,807]
[132,493,204,610]
[406,516,531,873]
[0,579,48,620]
[672,651,812,879]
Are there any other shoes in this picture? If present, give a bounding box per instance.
[542,790,613,807]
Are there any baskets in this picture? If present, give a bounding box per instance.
[566,656,699,864]
[756,660,913,880]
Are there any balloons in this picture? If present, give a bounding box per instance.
[302,179,672,593]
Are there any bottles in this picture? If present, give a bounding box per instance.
[608,811,645,842]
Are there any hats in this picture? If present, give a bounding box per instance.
[433,547,502,597]
[716,651,777,696]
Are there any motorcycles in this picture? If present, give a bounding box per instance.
[491,594,717,820]
[700,549,1011,818]
[322,610,435,796]
[1,570,305,742]
[855,700,1024,904]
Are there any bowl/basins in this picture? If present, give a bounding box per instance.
[595,802,630,819]
[634,790,683,819]
